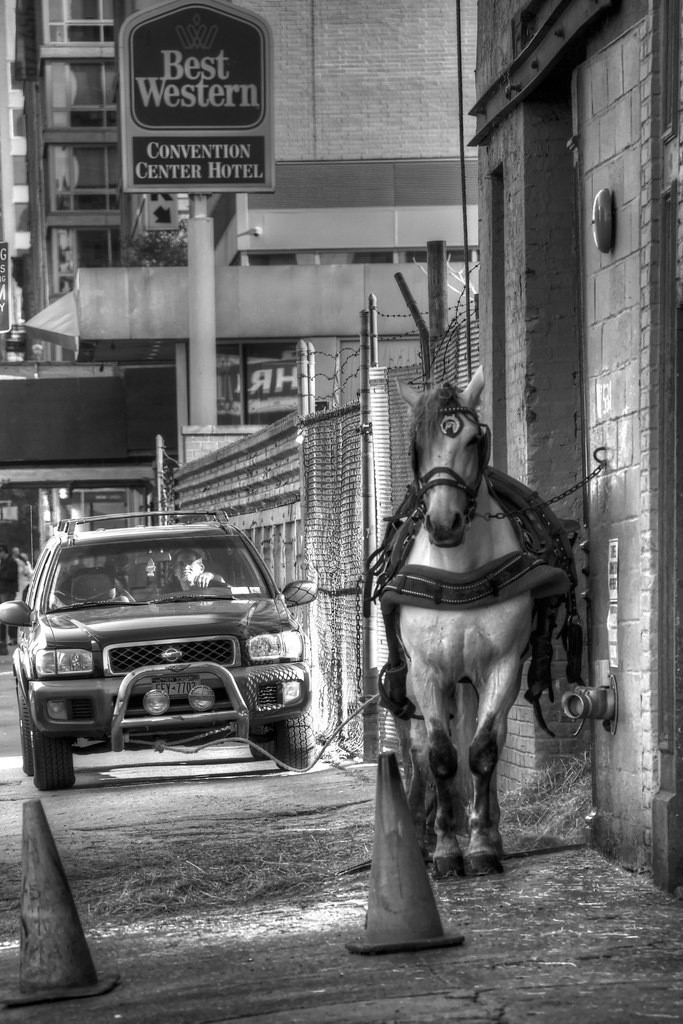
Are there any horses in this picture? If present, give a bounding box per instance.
[386,367,534,881]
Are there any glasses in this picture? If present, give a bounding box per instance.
[175,560,201,567]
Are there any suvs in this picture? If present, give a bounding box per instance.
[0,509,319,792]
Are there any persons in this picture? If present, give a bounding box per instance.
[0,545,32,656]
[157,547,229,595]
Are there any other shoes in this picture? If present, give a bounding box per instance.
[0,647,8,655]
[8,641,17,645]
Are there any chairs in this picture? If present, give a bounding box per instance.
[70,573,118,606]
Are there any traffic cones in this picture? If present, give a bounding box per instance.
[343,751,466,955]
[0,800,118,1007]
